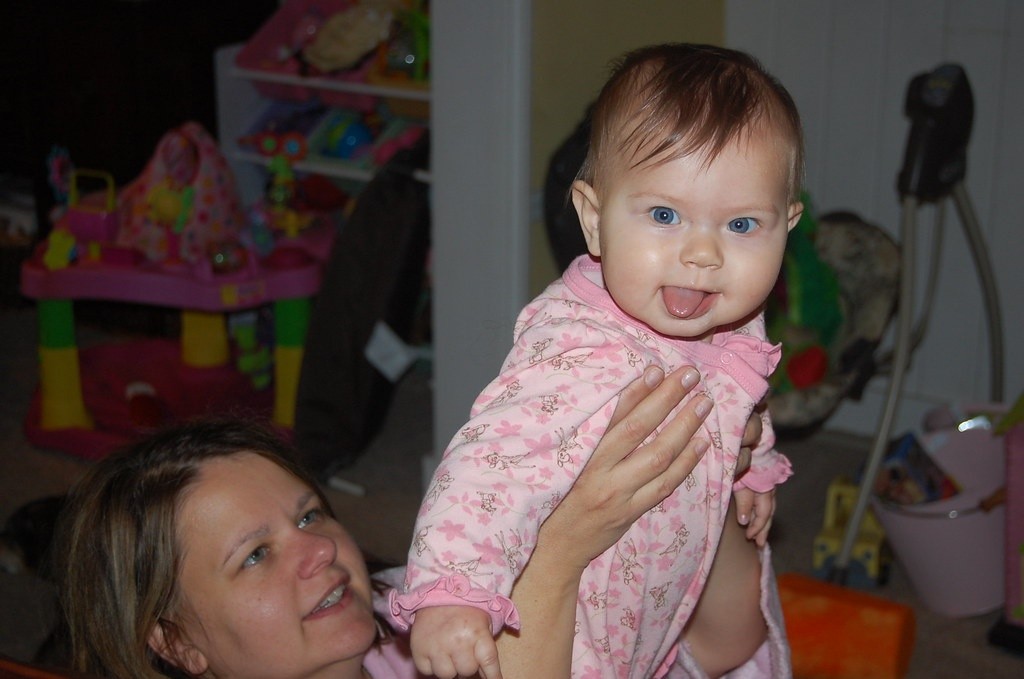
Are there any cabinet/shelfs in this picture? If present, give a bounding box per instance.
[215,44,433,367]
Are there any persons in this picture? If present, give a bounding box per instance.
[388,42,805,679]
[55,364,767,679]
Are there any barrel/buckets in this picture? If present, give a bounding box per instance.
[870,427,1005,616]
